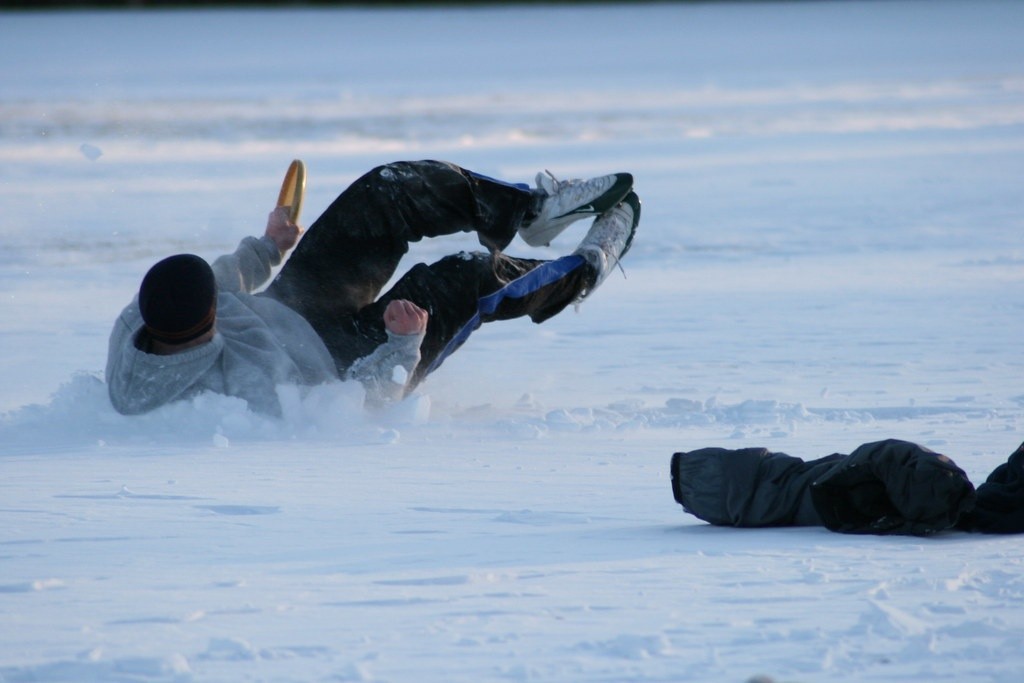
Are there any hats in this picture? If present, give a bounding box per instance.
[138,253,218,340]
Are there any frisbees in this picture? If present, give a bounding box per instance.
[275,158,307,225]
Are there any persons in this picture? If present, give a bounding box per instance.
[103,160,641,416]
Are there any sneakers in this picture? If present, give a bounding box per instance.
[571,191,642,305]
[517,168,634,247]
